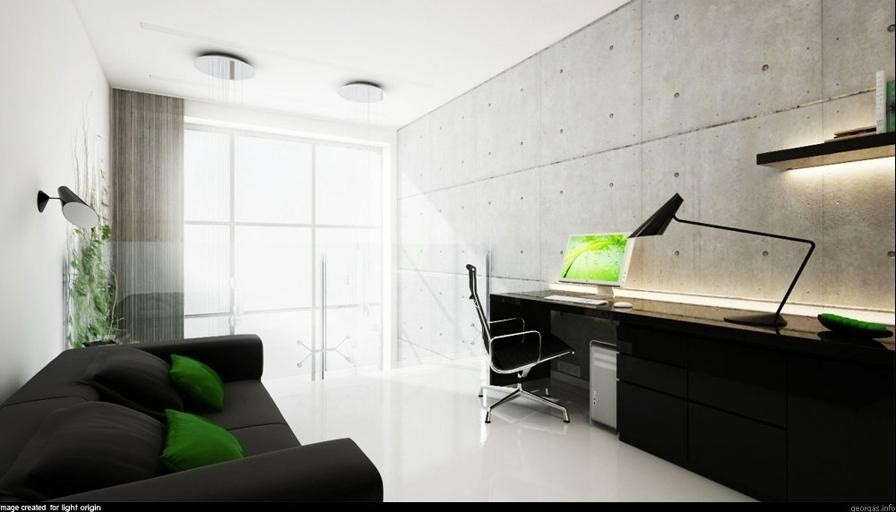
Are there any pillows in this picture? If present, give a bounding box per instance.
[169,353,227,410]
[0,402,167,505]
[97,344,184,415]
[160,408,244,472]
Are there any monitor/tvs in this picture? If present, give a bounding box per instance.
[558,232,634,299]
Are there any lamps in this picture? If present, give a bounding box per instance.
[36,185,100,233]
[625,192,816,326]
[191,52,254,81]
[337,81,386,104]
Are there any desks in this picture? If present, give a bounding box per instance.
[488,289,896,512]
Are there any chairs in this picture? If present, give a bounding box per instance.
[466,264,576,424]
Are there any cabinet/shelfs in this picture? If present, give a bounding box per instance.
[756,131,896,172]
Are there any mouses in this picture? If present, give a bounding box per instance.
[613,302,633,308]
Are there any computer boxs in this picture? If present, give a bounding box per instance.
[589,339,620,431]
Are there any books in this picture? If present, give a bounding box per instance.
[834,70,896,138]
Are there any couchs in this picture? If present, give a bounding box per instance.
[0,333,382,508]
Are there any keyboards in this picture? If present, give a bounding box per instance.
[544,295,608,305]
